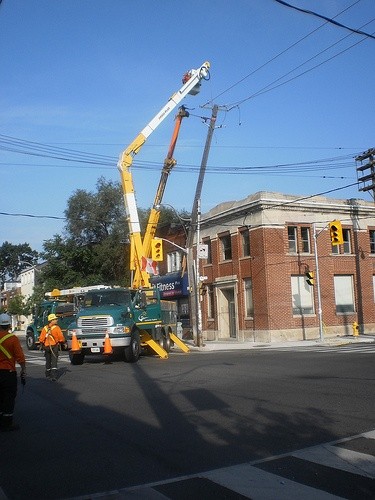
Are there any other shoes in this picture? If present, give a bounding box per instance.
[52,376,57,381]
[2,424,20,431]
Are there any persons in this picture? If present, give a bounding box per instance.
[0,314,26,431]
[39,313,68,381]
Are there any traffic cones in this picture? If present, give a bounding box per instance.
[68,328,84,367]
[100,332,114,364]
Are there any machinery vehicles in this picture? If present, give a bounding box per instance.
[26,284,117,351]
[63,60,212,366]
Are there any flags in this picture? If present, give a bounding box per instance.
[141,257,159,276]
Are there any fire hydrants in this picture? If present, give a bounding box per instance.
[352,320,360,337]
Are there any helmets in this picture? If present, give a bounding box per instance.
[0,314,12,325]
[47,314,59,322]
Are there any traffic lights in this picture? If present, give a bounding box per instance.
[329,220,343,246]
[305,271,314,286]
